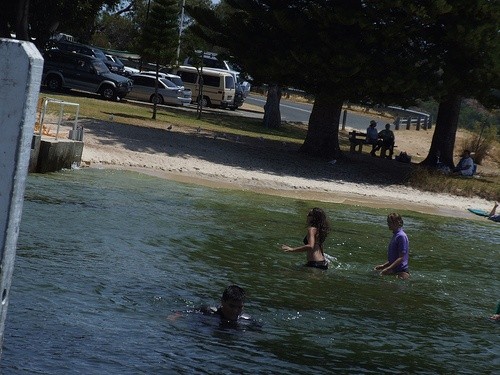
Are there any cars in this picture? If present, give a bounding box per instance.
[123,73,192,107]
[40,33,254,109]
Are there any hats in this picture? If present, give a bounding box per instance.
[369,120,377,126]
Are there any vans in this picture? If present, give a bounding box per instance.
[157,64,236,109]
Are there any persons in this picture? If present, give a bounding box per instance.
[202,285,251,320]
[378,123,395,159]
[282,207,329,269]
[430,150,473,175]
[375,213,409,276]
[367,120,382,157]
[488,201,500,221]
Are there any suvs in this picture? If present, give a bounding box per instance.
[42,49,134,102]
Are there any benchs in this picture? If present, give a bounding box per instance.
[348,130,397,153]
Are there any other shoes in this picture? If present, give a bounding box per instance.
[370,152,376,157]
